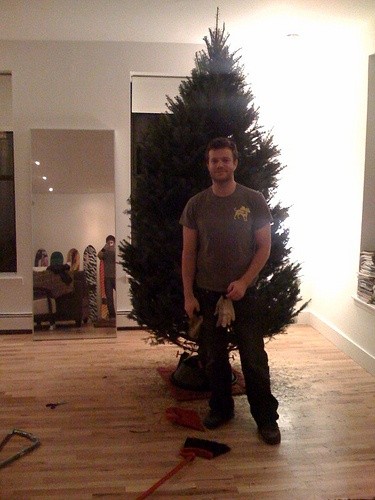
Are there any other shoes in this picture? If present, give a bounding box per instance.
[201,407,235,430]
[258,420,282,445]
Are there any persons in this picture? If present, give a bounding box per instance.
[180,137,282,445]
[97,235,116,324]
[47,252,70,271]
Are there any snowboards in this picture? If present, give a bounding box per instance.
[67,247,81,271]
[83,244,98,322]
[99,257,114,320]
[50,251,64,266]
[34,248,49,267]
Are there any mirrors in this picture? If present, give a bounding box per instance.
[30,129,118,340]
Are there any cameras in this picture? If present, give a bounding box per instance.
[110,241,113,246]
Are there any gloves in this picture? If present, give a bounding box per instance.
[214,294,236,328]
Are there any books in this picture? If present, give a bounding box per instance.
[357,250,375,305]
